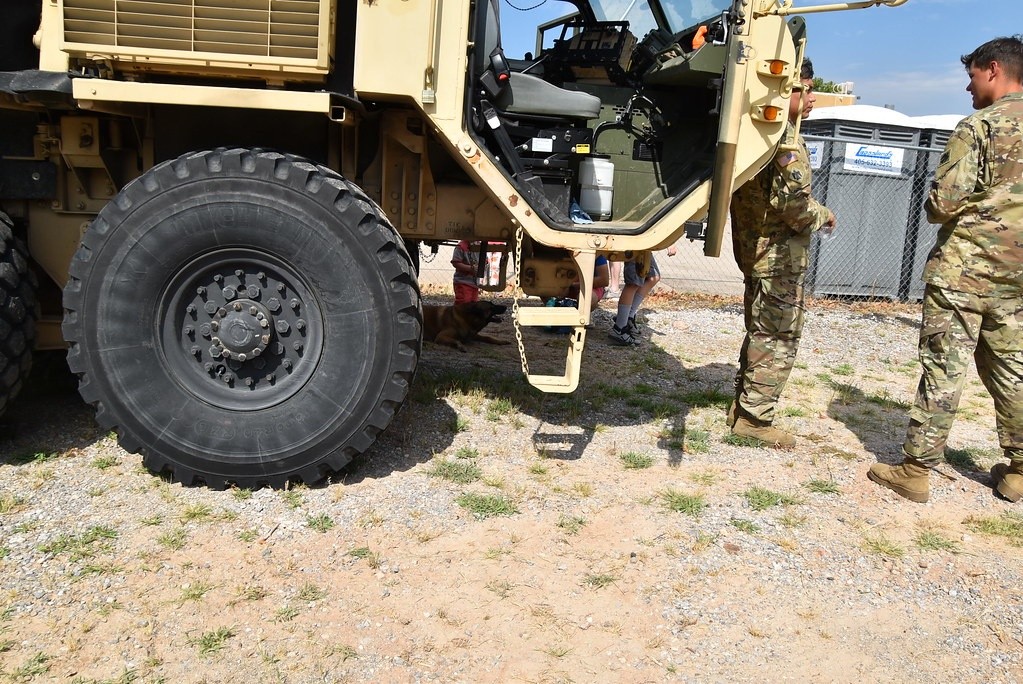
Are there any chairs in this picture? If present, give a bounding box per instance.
[474,0,601,120]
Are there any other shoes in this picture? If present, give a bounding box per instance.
[997,459,1023,503]
[726,398,744,428]
[732,407,797,448]
[587,312,595,328]
[867,454,931,503]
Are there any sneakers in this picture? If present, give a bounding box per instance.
[612,314,642,334]
[608,322,640,345]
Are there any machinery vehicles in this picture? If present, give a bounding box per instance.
[0,1,921,489]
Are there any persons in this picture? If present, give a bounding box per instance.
[609,244,676,346]
[868,37,1023,503]
[541,255,609,329]
[451,240,489,305]
[728,56,835,449]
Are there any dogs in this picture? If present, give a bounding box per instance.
[423,300,511,352]
